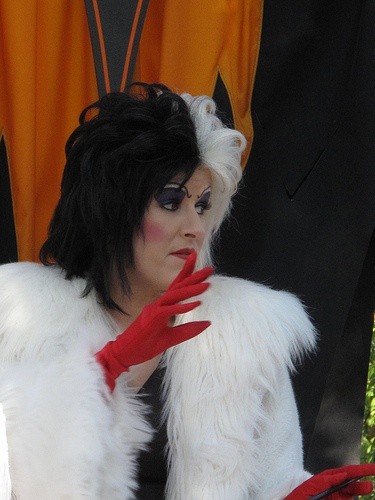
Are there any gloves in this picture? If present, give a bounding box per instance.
[282,463,375,500]
[92,252,214,393]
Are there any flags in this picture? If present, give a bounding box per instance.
[0,0,267,264]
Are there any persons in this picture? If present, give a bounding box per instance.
[1,82,374,500]
